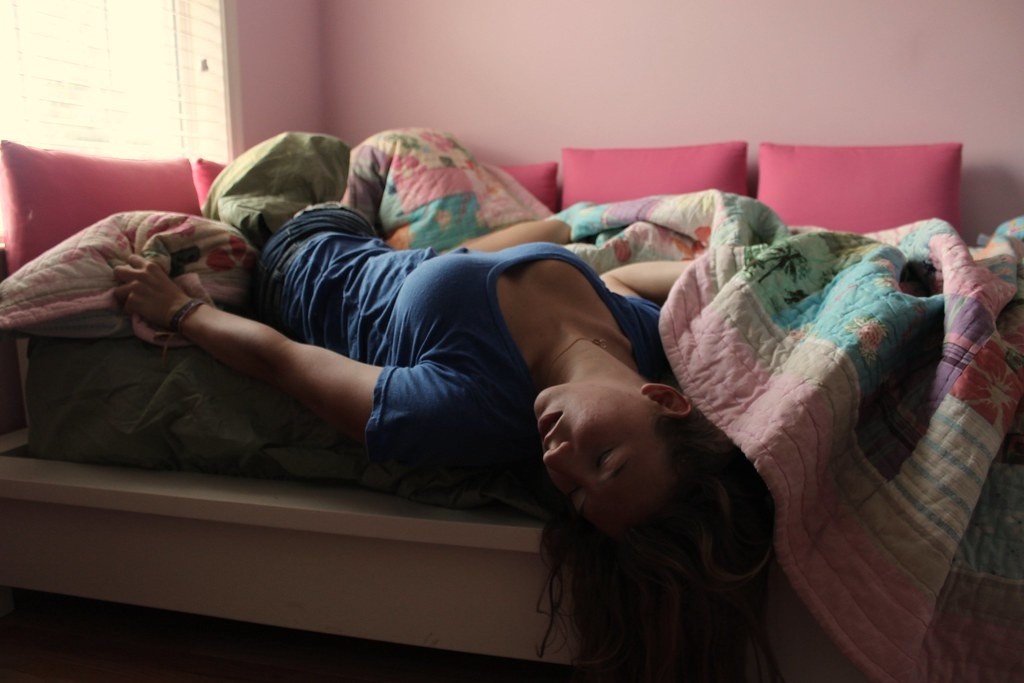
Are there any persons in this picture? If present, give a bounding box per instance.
[115,202,786,683]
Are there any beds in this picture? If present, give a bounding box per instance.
[0,128,1024,683]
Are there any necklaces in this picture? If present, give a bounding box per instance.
[542,337,609,380]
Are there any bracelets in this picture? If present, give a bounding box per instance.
[170,299,206,335]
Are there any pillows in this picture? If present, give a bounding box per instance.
[195,158,226,208]
[0,139,203,273]
[561,141,747,211]
[755,142,963,239]
[497,162,560,213]
